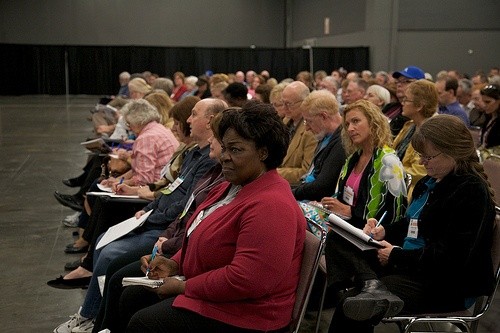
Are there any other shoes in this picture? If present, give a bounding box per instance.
[46,174,94,333]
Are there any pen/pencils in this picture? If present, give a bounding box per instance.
[117,144,121,148]
[368,211,387,237]
[146,245,158,275]
[119,176,124,184]
[111,142,114,148]
[331,192,340,198]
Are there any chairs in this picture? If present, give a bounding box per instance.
[381,206,500,333]
[290,217,327,333]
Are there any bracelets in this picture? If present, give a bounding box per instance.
[139,186,142,188]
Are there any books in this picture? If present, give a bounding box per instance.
[81,138,112,154]
[122,276,185,288]
[328,213,379,245]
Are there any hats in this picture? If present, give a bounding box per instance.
[480,84,500,100]
[392,66,424,78]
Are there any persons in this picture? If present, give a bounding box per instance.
[46,66,500,333]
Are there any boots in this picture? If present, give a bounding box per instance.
[342,280,403,320]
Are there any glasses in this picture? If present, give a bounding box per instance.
[271,103,283,107]
[394,79,414,84]
[283,100,303,107]
[401,97,415,105]
